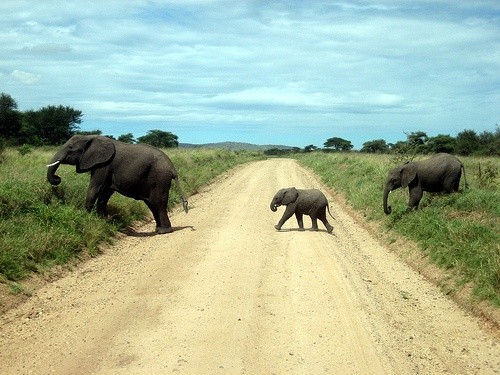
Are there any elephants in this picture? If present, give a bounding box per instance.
[383,153,468,215]
[270,187,336,233]
[46,134,188,234]
